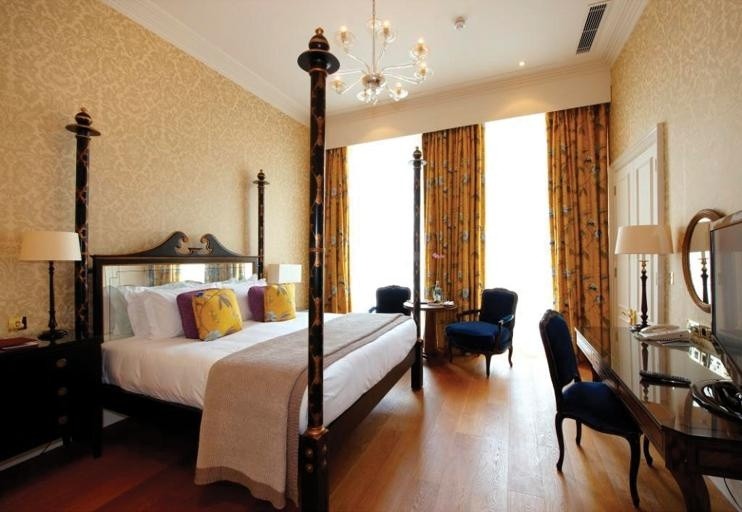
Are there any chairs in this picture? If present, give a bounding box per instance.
[365,285,412,321]
[538,309,654,510]
[444,287,519,379]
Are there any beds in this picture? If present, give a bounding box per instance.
[65,27,428,510]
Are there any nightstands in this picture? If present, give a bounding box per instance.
[297,303,309,312]
[0,331,104,490]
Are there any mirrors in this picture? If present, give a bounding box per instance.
[679,208,725,314]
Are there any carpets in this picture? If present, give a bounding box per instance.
[94,465,258,509]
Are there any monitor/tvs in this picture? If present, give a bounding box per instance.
[691,210,742,424]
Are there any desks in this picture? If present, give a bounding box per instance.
[402,299,459,362]
[573,322,741,511]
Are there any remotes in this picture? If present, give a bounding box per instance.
[639,369,691,387]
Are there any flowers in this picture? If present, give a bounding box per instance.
[432,252,447,287]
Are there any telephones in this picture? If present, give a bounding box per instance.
[634,323,692,342]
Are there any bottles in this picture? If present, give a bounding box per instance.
[433,281,441,302]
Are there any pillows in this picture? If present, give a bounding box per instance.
[118,281,189,339]
[207,274,258,285]
[137,282,223,340]
[177,286,220,338]
[246,284,269,322]
[215,278,269,322]
[191,287,244,341]
[262,282,297,323]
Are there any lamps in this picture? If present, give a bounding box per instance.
[688,220,715,304]
[613,224,676,334]
[264,262,303,287]
[328,1,432,109]
[18,230,86,339]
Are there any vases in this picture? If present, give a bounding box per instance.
[433,285,442,302]
